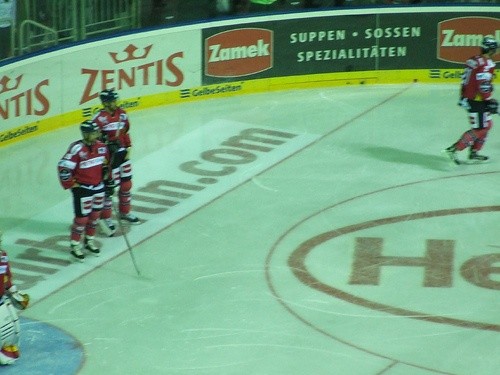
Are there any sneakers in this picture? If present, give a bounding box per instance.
[83,234,101,256]
[69,239,85,263]
[102,218,117,229]
[119,213,141,224]
[467,151,490,163]
[442,146,460,167]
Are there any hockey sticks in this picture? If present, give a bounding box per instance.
[110,197,143,275]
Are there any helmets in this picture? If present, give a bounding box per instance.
[81,120,100,132]
[100,88,120,102]
[480,34,500,50]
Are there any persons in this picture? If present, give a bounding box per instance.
[92,89,143,234]
[56,121,114,260]
[0,248,22,367]
[444,37,499,164]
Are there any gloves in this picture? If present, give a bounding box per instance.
[486,100,498,114]
[4,284,29,310]
